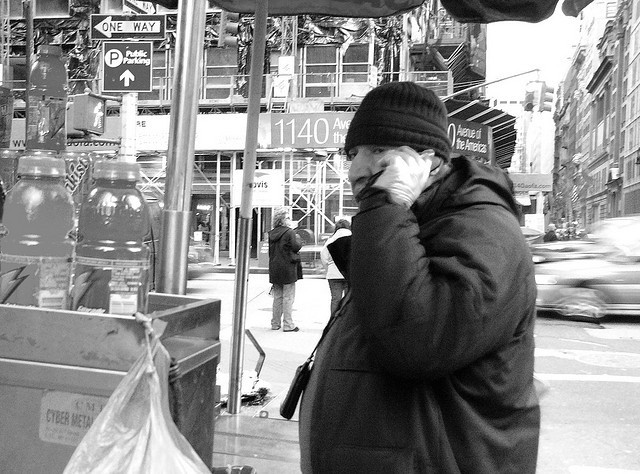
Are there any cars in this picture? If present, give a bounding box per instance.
[579,216,640,255]
[529,239,640,322]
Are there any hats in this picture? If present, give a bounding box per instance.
[344,81,452,163]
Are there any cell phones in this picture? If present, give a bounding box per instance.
[418,148,441,173]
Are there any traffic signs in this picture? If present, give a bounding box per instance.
[90,14,166,41]
[102,41,153,92]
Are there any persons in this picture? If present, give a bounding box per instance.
[543,222,559,241]
[279,83,539,473]
[320,220,352,320]
[267,206,304,333]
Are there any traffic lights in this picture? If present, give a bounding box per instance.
[73,93,106,136]
[539,83,554,112]
[523,90,534,111]
[217,10,241,49]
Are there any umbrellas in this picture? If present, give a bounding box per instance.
[136,0,596,414]
